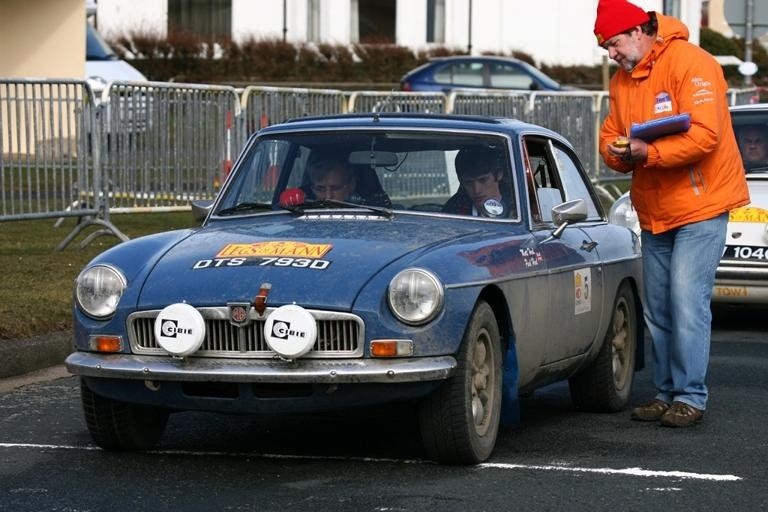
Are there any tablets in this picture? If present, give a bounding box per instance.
[630,112,690,143]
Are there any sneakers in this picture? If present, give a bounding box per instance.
[657,400,705,429]
[630,399,670,423]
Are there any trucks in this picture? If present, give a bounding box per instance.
[85,0,158,139]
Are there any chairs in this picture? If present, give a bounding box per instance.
[305,161,392,213]
[453,176,512,206]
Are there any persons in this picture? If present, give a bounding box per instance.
[301,156,364,204]
[737,127,768,165]
[594,0,750,426]
[441,145,516,220]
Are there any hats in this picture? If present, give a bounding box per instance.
[593,0,651,46]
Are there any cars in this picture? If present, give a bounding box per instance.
[397,51,593,141]
[56,108,652,472]
[599,100,767,312]
